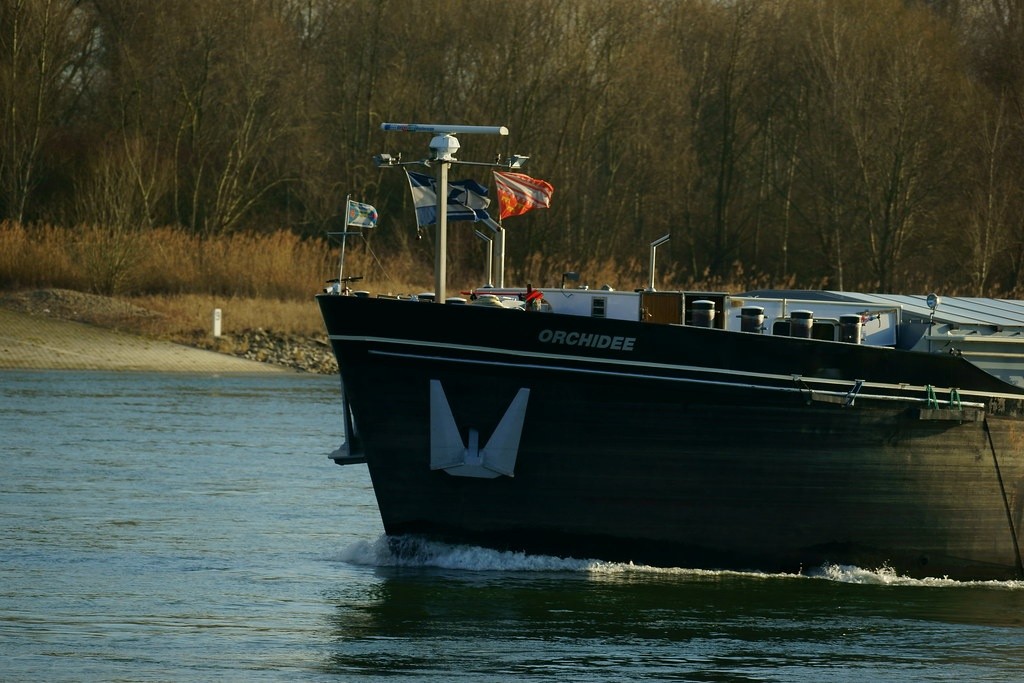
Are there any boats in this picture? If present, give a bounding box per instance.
[315,125,1024,584]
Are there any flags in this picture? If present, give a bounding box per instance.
[347,200,378,228]
[494,170,554,219]
[406,171,489,229]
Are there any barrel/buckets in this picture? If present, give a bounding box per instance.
[839,314,861,345]
[741,306,764,334]
[790,310,814,338]
[691,299,715,328]
